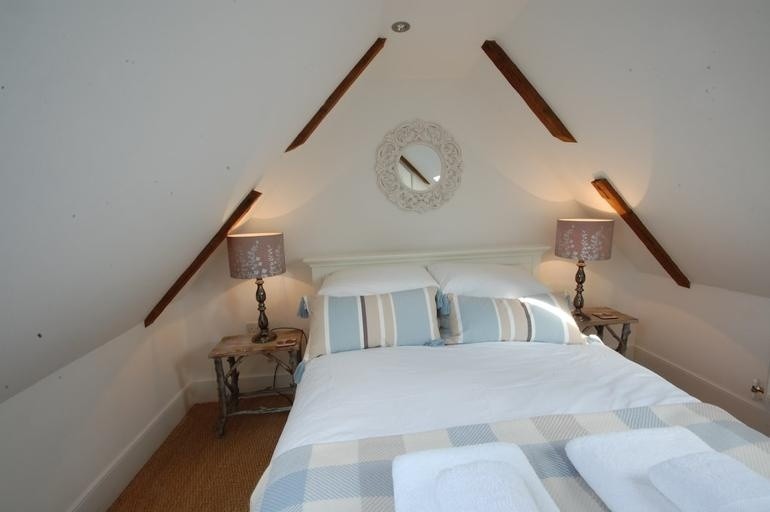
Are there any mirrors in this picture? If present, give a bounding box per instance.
[374,118,462,214]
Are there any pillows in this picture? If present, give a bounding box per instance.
[440,288,588,348]
[299,284,447,361]
[426,259,552,302]
[316,262,443,308]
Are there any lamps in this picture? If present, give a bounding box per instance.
[554,218,615,322]
[225,232,287,346]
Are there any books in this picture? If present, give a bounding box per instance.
[592,312,618,319]
[275,334,298,347]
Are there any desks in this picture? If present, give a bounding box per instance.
[208,332,304,439]
[574,307,639,357]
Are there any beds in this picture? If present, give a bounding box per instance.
[252,242,770,510]
